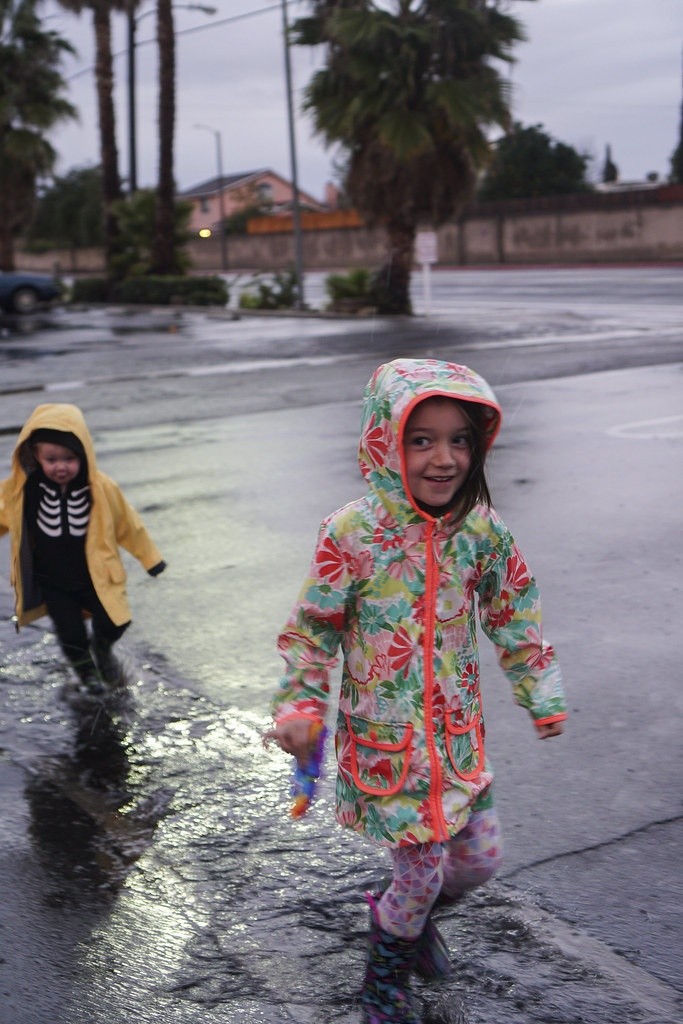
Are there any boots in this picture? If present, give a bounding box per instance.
[416,895,463,979]
[360,908,422,1024]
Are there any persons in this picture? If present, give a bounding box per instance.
[1,401,170,694]
[266,357,571,1023]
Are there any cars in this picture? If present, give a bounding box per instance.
[0,268,62,314]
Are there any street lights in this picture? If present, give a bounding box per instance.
[124,4,220,194]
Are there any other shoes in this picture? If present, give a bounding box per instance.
[81,670,106,697]
[94,644,122,688]
